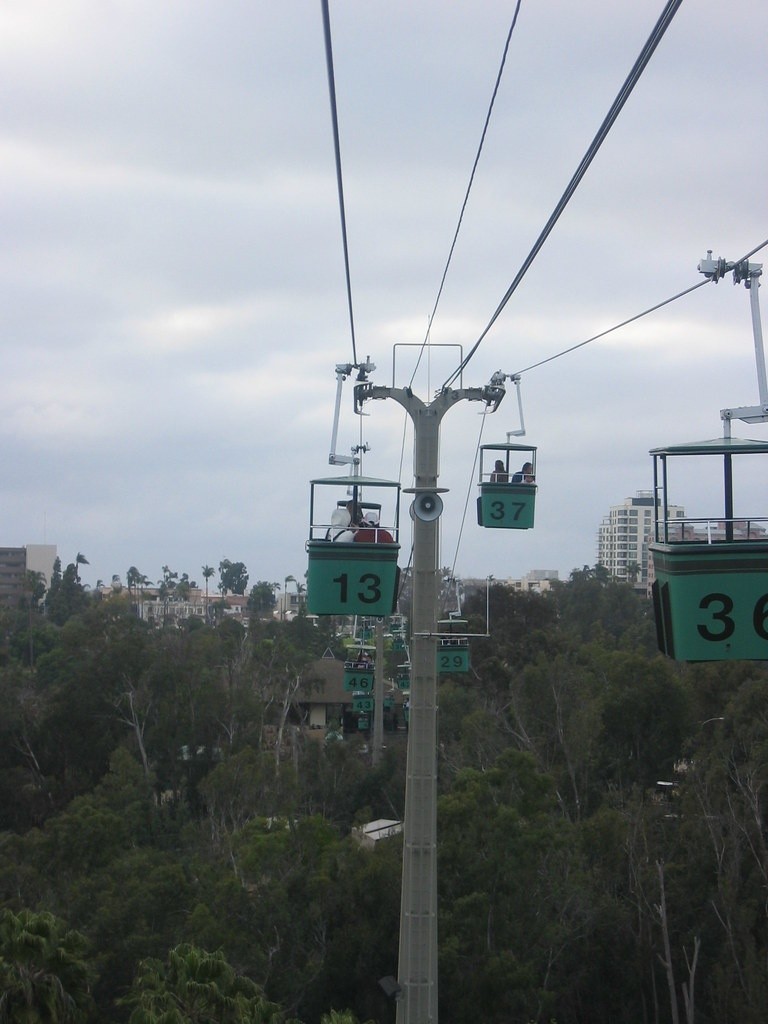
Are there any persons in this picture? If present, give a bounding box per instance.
[403,698,409,707]
[490,459,506,482]
[330,500,394,543]
[356,655,372,668]
[512,462,534,483]
[440,630,458,644]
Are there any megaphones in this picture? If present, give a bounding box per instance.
[376,617,383,623]
[414,492,443,522]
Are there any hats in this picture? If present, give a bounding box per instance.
[363,512,379,526]
